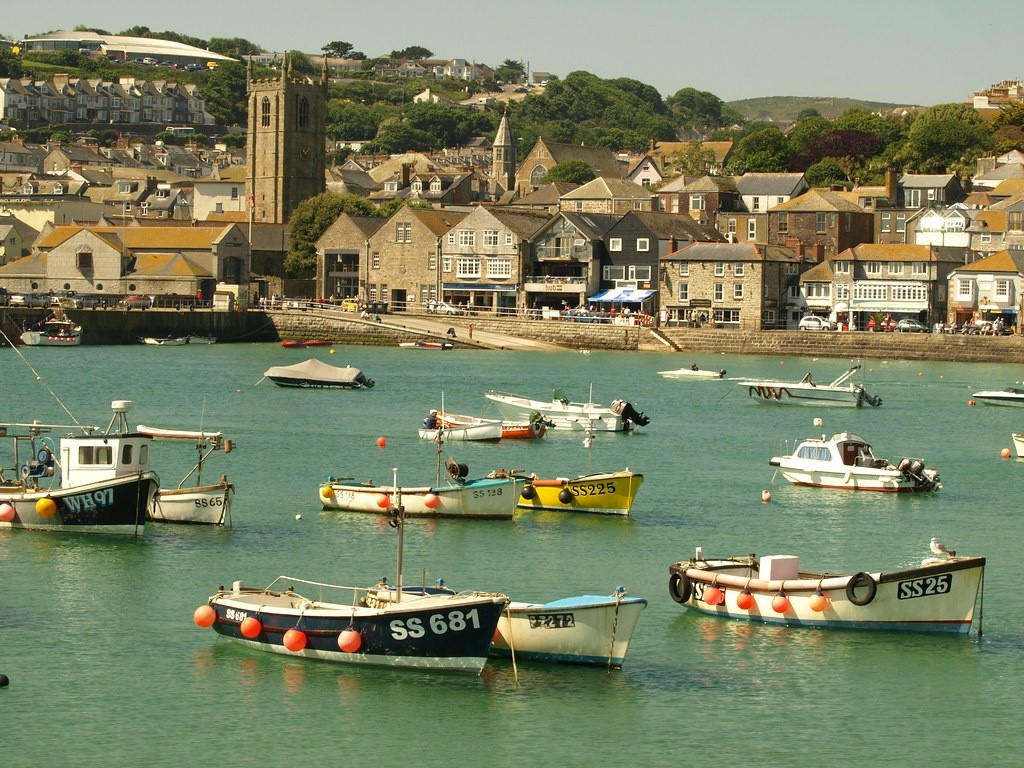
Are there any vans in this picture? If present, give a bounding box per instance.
[797,315,837,331]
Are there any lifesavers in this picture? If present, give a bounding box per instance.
[77,302,84,309]
[190,306,194,311]
[102,303,106,308]
[44,301,49,309]
[846,572,877,606]
[93,303,97,310]
[27,301,34,309]
[668,571,691,604]
[610,400,621,413]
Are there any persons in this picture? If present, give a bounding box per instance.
[962,317,1004,336]
[686,311,714,328]
[330,294,334,303]
[272,293,283,307]
[254,291,259,303]
[318,297,325,308]
[950,323,958,335]
[309,297,316,306]
[665,310,671,327]
[361,310,381,323]
[469,325,472,339]
[938,320,944,334]
[562,302,645,323]
[467,300,470,309]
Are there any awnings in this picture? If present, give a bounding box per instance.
[848,301,924,313]
[587,288,658,313]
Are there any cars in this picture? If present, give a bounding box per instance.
[71,294,100,310]
[117,296,152,310]
[10,292,51,309]
[426,303,464,316]
[51,290,78,307]
[0,288,12,308]
[893,319,930,334]
[341,298,361,310]
[974,320,1014,336]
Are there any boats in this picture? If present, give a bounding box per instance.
[398,341,456,350]
[280,336,336,348]
[768,430,944,495]
[971,384,1024,409]
[191,490,511,677]
[141,398,238,528]
[186,334,218,345]
[143,337,189,346]
[262,357,377,390]
[417,420,505,445]
[443,456,647,517]
[317,428,529,520]
[0,399,162,538]
[136,424,225,440]
[1010,431,1024,459]
[425,408,559,440]
[737,360,883,409]
[664,534,989,638]
[355,576,649,670]
[654,366,727,380]
[18,307,84,347]
[485,381,651,433]
